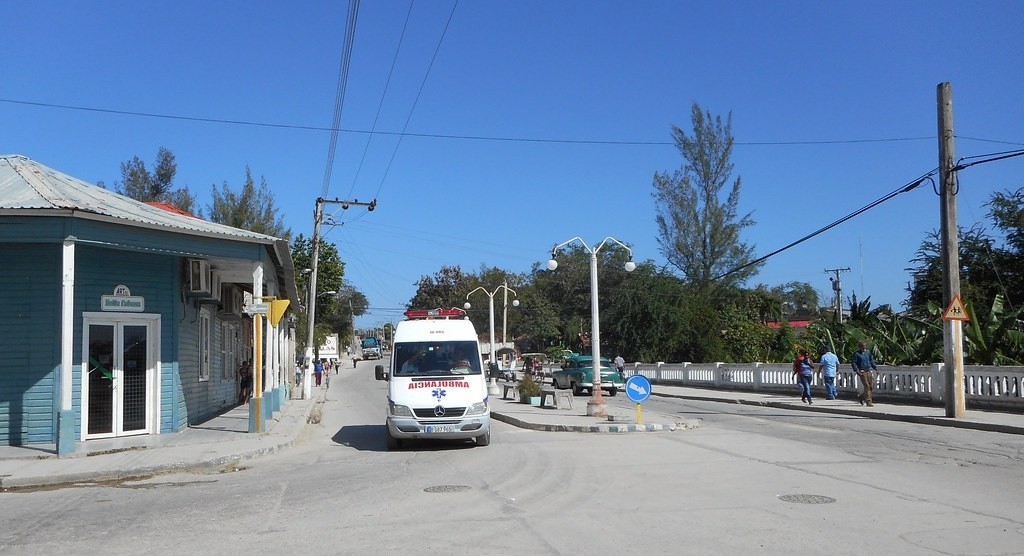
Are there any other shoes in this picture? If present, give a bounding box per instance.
[859,398,864,406]
[826,397,830,400]
[867,403,873,406]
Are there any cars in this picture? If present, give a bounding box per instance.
[561,350,579,357]
[551,356,625,397]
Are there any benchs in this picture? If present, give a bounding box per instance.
[540,388,574,412]
[503,383,520,401]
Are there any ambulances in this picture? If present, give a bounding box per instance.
[375,309,491,448]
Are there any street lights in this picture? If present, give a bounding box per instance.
[305,289,336,403]
[463,284,519,396]
[548,236,637,418]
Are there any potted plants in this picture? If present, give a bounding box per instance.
[517,372,542,403]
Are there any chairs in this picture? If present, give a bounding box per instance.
[397,352,413,372]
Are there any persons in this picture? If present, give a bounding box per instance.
[239,345,358,407]
[817,346,839,400]
[851,342,880,406]
[499,356,539,377]
[791,350,814,405]
[614,353,626,372]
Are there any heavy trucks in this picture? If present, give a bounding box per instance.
[361,337,384,361]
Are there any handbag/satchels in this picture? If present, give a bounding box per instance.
[245,376,250,387]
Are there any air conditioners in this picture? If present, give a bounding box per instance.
[217,284,242,320]
[192,271,222,323]
[182,259,211,322]
